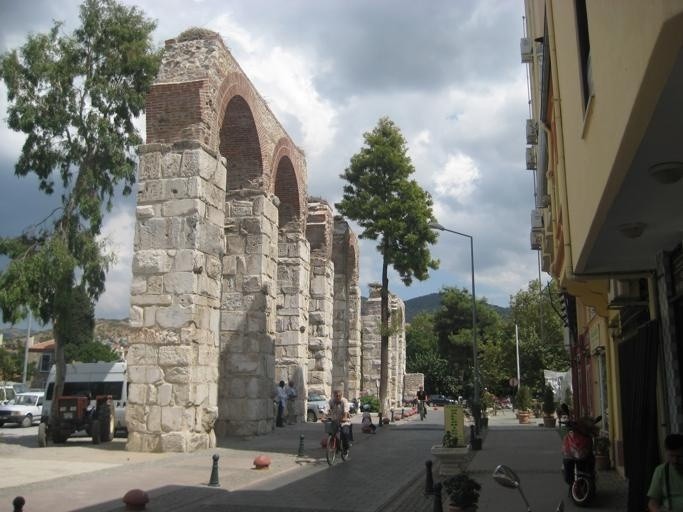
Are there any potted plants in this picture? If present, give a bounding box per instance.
[477,402,489,431]
[516,383,532,423]
[432,430,471,475]
[541,382,556,427]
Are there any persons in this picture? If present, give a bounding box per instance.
[286,381,297,425]
[415,387,427,418]
[646,434,683,512]
[275,380,285,427]
[320,390,353,456]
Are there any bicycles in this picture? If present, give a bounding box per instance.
[323,388,357,467]
[414,382,430,421]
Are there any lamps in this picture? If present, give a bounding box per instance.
[647,162,681,184]
[618,222,644,239]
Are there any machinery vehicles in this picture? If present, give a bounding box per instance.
[38,394,115,448]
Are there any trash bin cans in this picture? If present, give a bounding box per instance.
[473,438,482,450]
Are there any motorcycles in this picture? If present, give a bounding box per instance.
[555,402,603,506]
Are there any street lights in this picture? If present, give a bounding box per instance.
[429,219,483,449]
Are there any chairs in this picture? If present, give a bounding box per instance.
[556,408,576,431]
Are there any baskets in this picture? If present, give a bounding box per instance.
[325,422,339,433]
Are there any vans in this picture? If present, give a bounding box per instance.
[45,363,129,430]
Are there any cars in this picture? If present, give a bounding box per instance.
[307,392,356,422]
[427,394,459,406]
[0,386,44,426]
